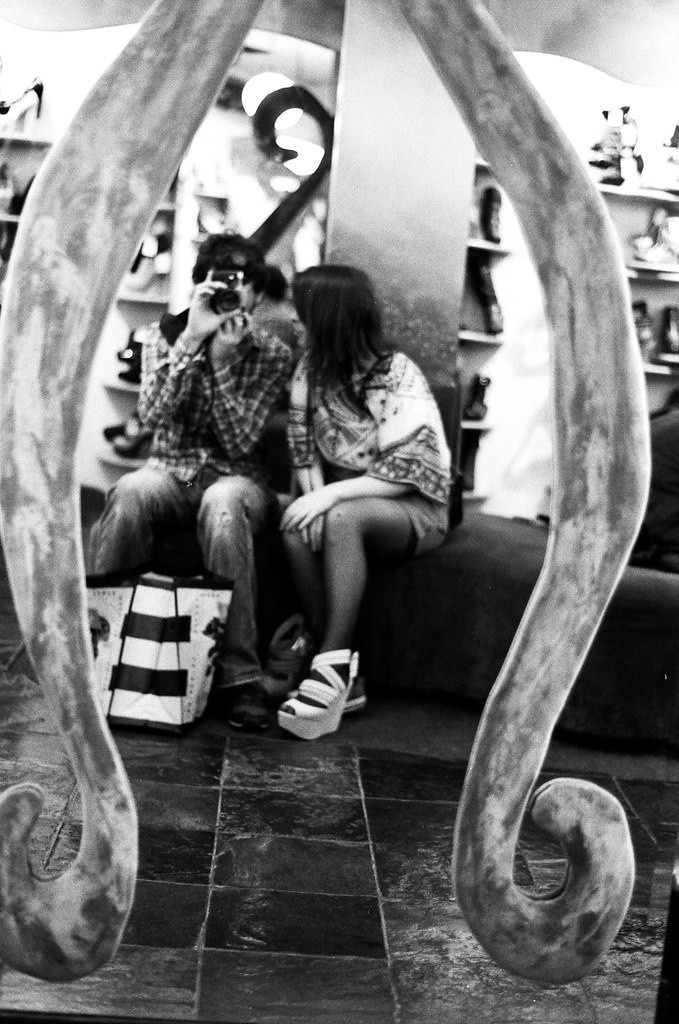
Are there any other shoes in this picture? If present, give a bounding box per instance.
[223,682,275,735]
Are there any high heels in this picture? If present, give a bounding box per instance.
[267,612,367,740]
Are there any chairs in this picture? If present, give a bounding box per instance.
[381,504,679,752]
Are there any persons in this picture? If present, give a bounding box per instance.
[277,264,452,743]
[91,226,291,730]
[245,263,303,350]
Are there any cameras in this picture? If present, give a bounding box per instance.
[210,270,242,315]
